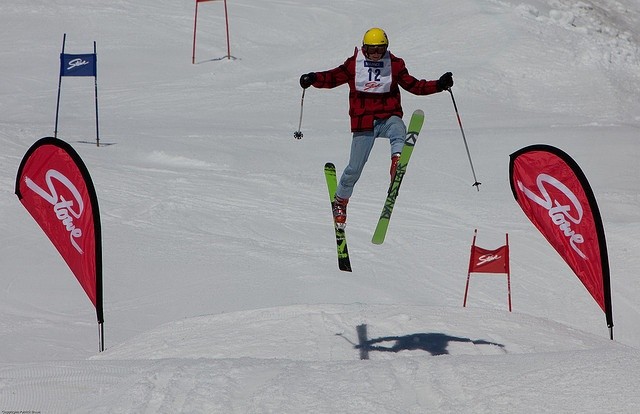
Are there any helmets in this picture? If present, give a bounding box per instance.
[364,28,388,46]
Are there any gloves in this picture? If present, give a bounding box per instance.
[436,72,453,92]
[300,72,316,88]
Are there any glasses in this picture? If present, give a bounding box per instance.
[368,48,386,54]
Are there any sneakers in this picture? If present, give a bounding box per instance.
[334,192,349,223]
[390,156,401,182]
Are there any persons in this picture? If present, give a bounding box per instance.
[299,26,453,223]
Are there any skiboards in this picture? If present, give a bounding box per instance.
[324,109,426,273]
